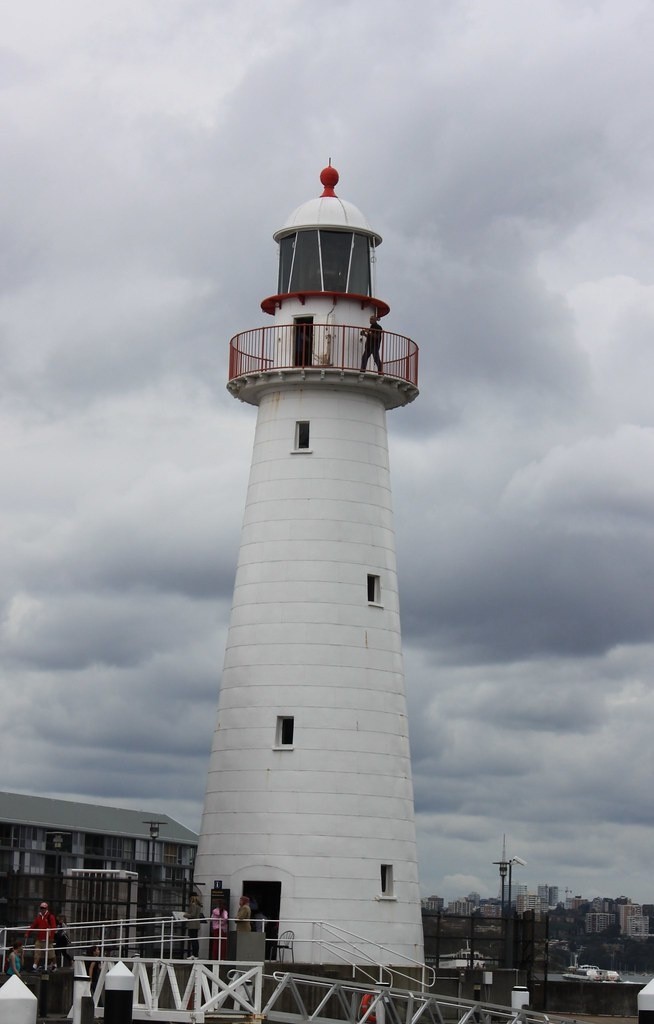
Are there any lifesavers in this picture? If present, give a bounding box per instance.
[360,993,377,1022]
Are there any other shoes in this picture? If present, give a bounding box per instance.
[187,955,198,960]
[26,967,36,973]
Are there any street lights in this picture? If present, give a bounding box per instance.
[46,830,71,914]
[492,862,509,935]
[141,821,168,918]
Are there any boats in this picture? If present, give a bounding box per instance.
[562,964,622,982]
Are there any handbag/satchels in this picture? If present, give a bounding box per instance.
[4,957,9,971]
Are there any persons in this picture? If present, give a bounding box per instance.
[183,892,203,960]
[360,316,384,375]
[234,896,251,932]
[7,940,24,976]
[84,946,103,1007]
[211,903,228,960]
[24,902,57,973]
[54,914,74,969]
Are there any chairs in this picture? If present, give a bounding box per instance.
[269,929,294,965]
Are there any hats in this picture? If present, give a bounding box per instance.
[240,895,249,906]
[40,902,48,908]
[188,892,197,897]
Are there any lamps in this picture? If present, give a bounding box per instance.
[150,822,159,838]
